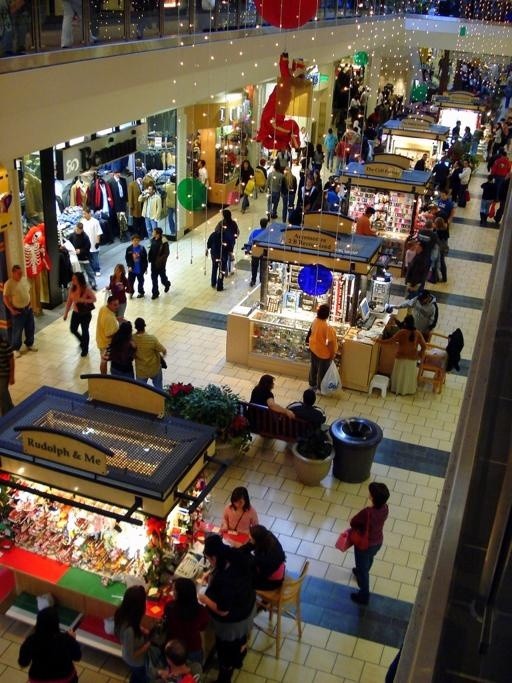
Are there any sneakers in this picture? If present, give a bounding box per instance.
[27,346,38,352]
[13,351,21,357]
[137,294,145,298]
[350,591,369,604]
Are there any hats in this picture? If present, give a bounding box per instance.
[417,288,428,300]
[369,481,390,502]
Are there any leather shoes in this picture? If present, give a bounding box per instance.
[164,281,171,292]
[152,291,159,299]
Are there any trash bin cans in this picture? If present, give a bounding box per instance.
[329,417,383,483]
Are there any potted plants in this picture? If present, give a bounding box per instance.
[163,382,245,464]
[292,426,336,487]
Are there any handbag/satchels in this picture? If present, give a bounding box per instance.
[252,178,258,199]
[489,202,497,218]
[244,180,255,196]
[144,616,167,648]
[335,528,353,551]
[103,346,111,362]
[227,190,239,205]
[349,506,371,551]
[77,301,94,313]
[305,329,311,343]
[439,241,449,256]
[435,259,441,269]
[466,191,470,202]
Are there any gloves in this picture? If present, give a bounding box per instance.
[95,243,99,249]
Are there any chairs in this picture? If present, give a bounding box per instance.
[417,328,460,394]
[248,560,312,660]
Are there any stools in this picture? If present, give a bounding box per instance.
[368,374,390,399]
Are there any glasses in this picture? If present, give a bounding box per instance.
[134,239,140,242]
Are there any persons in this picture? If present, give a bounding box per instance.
[481,116,511,225]
[331,62,411,162]
[1,1,158,57]
[425,55,512,119]
[64,207,170,391]
[1,339,16,417]
[403,121,484,299]
[17,608,81,682]
[317,2,512,23]
[2,265,38,356]
[196,127,352,289]
[113,487,288,682]
[308,303,338,393]
[349,482,391,607]
[107,171,147,241]
[357,206,377,235]
[249,374,326,452]
[138,175,177,247]
[372,290,438,396]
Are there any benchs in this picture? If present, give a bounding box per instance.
[236,399,312,444]
